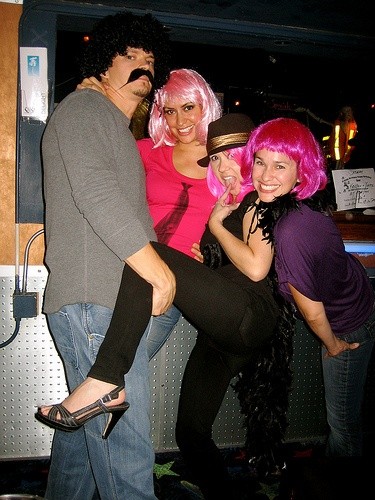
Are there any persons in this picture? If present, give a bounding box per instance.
[72,66,231,370]
[34,111,280,500]
[40,9,177,500]
[240,117,375,457]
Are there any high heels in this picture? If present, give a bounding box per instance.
[34,383,129,440]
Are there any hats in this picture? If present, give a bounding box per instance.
[195,113,256,166]
